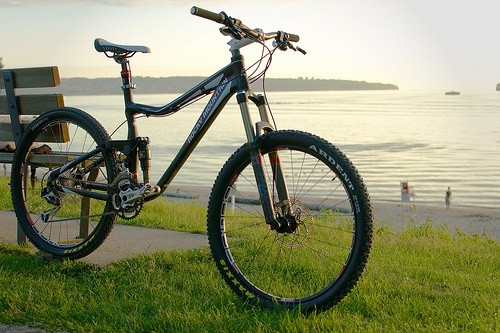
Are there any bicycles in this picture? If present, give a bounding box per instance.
[10,4,374,312]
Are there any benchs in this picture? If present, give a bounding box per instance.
[0,66,126,245]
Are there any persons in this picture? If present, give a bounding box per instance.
[445,186,452,211]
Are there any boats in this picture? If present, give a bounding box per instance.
[446,91,460,95]
